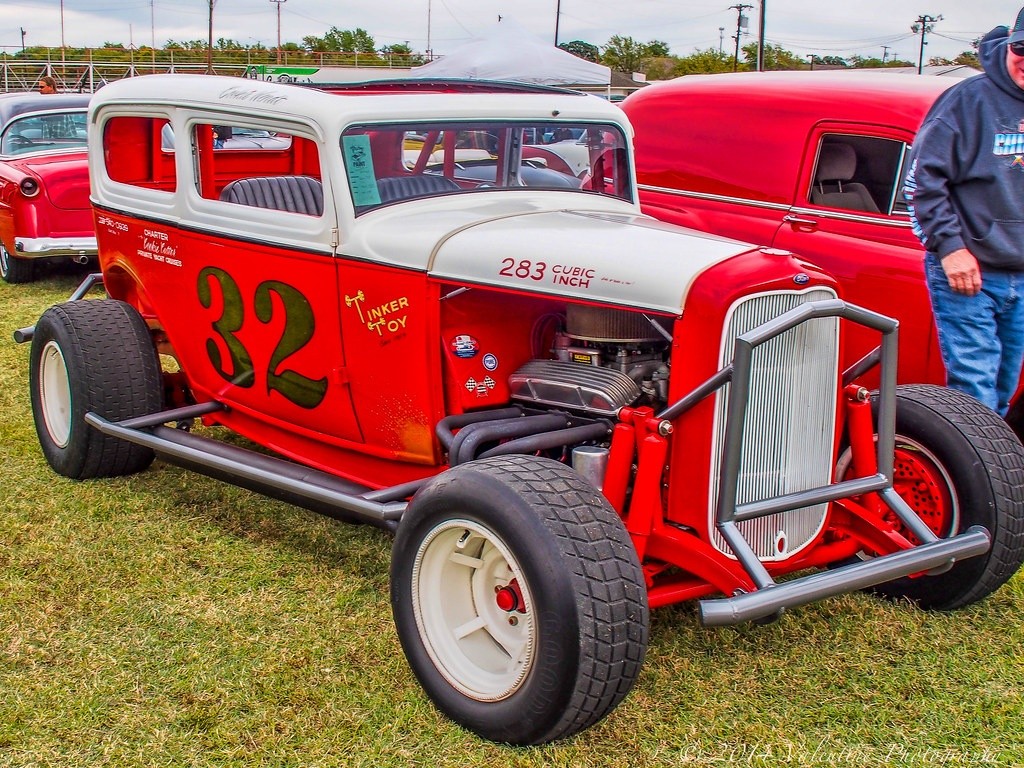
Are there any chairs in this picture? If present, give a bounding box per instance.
[219,176,325,217]
[356,175,462,216]
[810,143,881,215]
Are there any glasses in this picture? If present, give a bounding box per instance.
[1009,41,1023,56]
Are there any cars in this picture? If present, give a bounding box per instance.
[579,66,1024,443]
[15,72,1024,746]
[0,90,591,283]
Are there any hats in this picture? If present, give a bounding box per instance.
[1006,6,1024,44]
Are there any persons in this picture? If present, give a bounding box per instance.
[38,76,79,138]
[212,125,232,149]
[902,6,1024,416]
[549,128,573,144]
